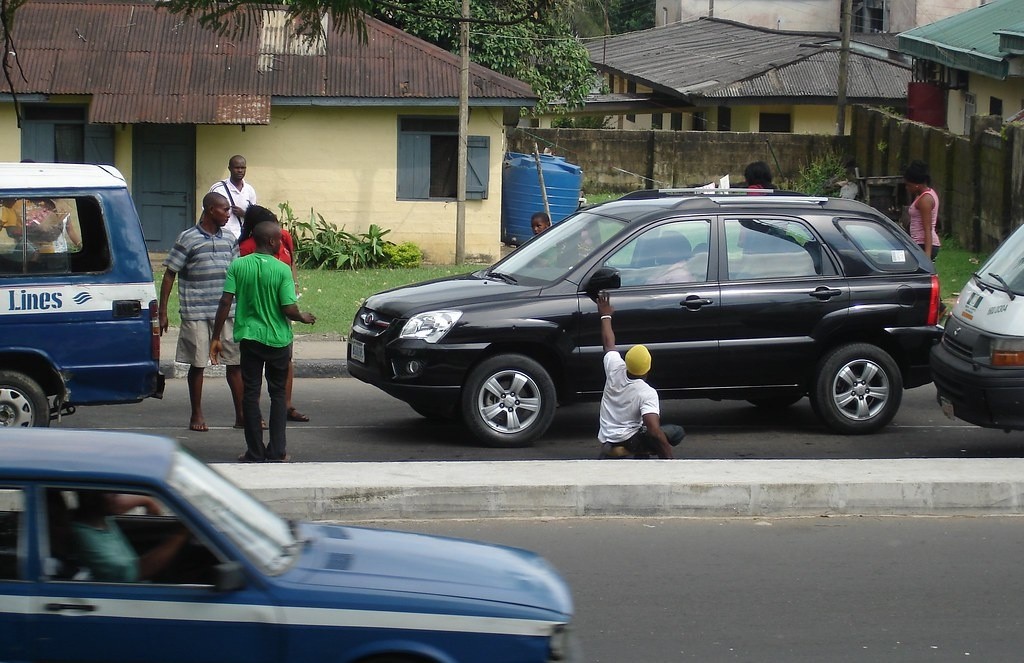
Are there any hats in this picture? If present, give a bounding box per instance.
[625,345,651,375]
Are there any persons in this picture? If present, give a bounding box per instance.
[530,213,559,268]
[0,158,84,254]
[201,154,258,240]
[903,160,947,323]
[209,221,317,463]
[158,192,269,431]
[739,161,788,254]
[596,289,685,460]
[236,204,310,429]
[69,487,196,584]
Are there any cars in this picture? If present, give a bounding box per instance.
[0,426,576,662]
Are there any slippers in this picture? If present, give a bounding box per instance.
[190,422,209,432]
[233,426,244,429]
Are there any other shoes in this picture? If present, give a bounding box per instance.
[939,307,947,321]
[284,454,291,461]
[239,454,251,461]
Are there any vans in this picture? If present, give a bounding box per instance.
[0,161,169,427]
[928,222,1024,436]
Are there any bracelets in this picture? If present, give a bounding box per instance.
[294,283,300,287]
[77,242,81,247]
[600,315,612,321]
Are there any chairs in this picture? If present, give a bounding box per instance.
[45,491,68,549]
[656,231,692,263]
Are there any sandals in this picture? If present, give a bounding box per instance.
[261,418,268,429]
[287,407,310,422]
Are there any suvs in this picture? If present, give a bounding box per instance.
[344,188,950,446]
